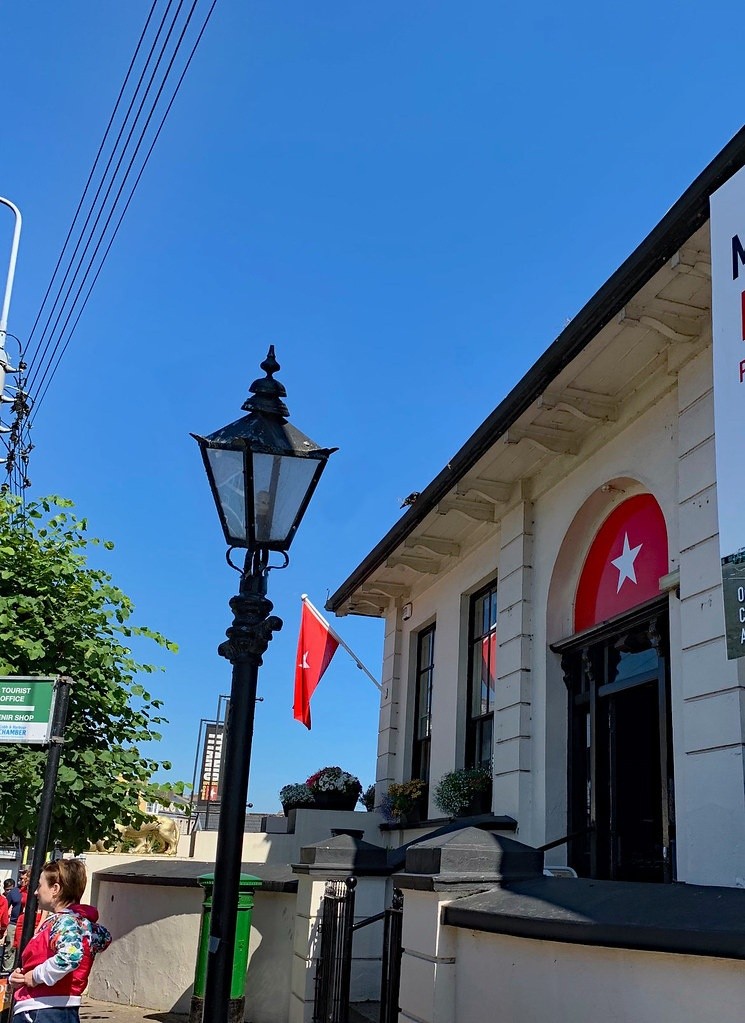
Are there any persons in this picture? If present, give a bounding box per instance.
[0,869,49,973]
[482,633,497,694]
[8,858,99,1023]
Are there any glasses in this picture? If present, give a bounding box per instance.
[51,857,65,885]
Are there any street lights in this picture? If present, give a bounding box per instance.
[186,341,340,1023]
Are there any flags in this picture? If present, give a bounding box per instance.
[292,604,341,730]
